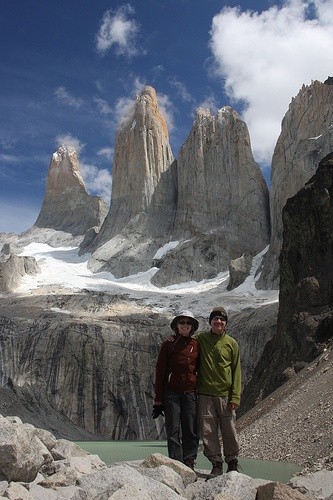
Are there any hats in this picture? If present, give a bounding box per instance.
[170,310,199,337]
[209,306,227,327]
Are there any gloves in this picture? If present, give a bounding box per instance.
[151,403,164,419]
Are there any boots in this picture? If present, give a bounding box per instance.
[207,462,223,479]
[226,458,244,473]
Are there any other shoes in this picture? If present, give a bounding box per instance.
[183,457,197,471]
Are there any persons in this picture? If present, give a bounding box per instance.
[165,307,242,480]
[154,309,202,472]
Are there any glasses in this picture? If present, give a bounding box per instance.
[213,317,225,321]
[176,321,192,325]
